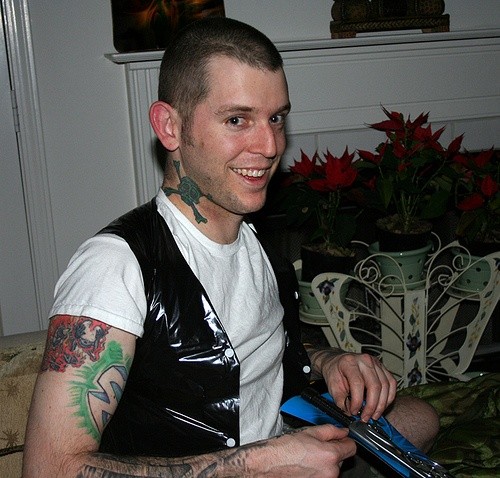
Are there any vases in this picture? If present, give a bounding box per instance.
[450,231,500,290]
[365,213,434,283]
[294,240,357,315]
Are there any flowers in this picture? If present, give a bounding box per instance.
[459,145,500,227]
[275,147,362,249]
[358,103,464,229]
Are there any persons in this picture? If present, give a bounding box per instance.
[22,17,439,478]
